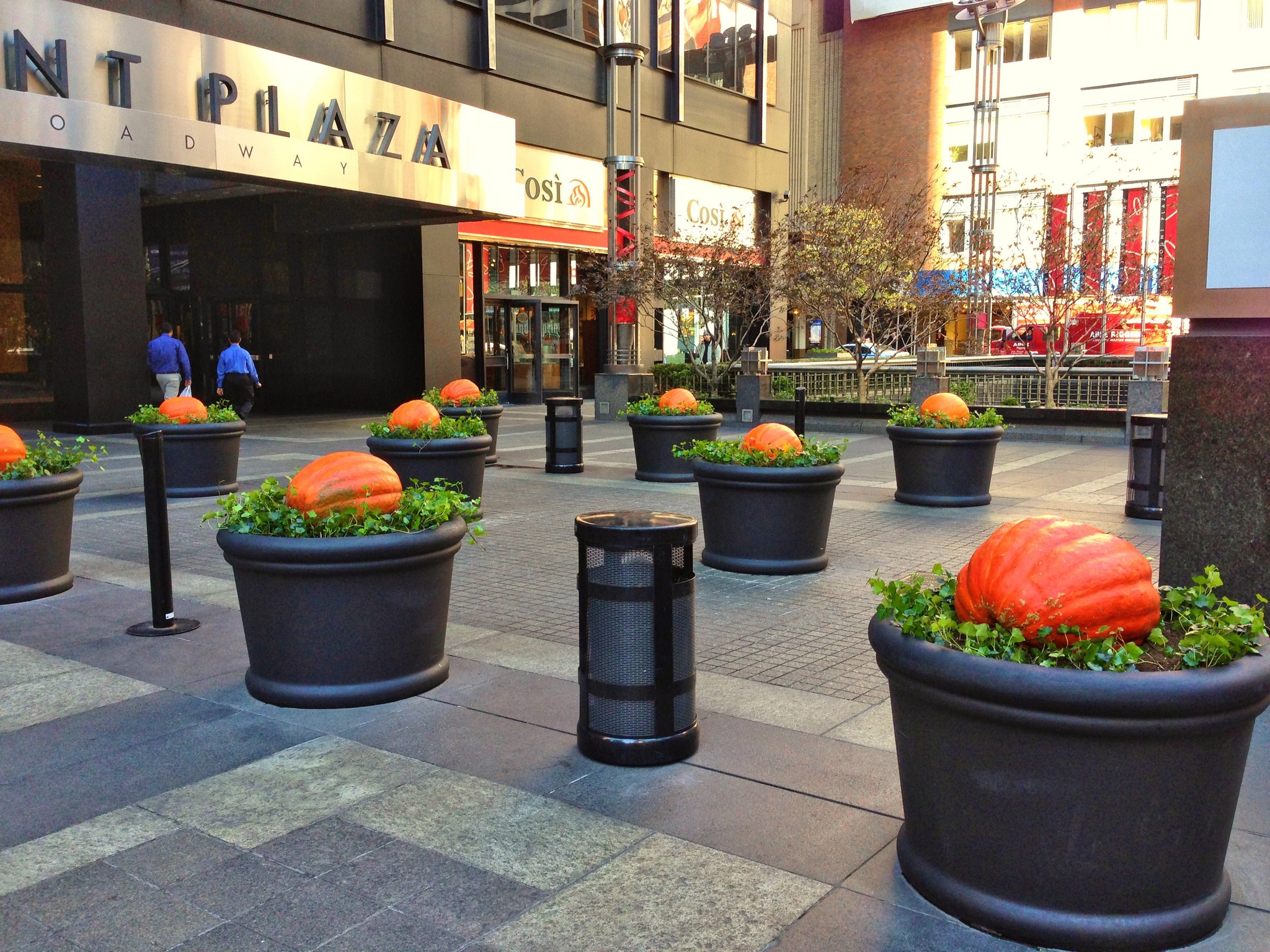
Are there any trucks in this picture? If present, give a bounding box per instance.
[984,314,1172,355]
[985,326,1010,341]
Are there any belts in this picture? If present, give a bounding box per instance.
[156,372,179,374]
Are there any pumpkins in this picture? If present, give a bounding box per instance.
[953,515,1160,652]
[920,393,970,428]
[158,396,208,424]
[285,451,402,527]
[658,388,697,415]
[0,424,27,474]
[440,379,481,408]
[388,400,440,433]
[739,423,802,464]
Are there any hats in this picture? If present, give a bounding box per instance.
[703,331,709,336]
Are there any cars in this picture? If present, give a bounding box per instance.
[835,339,911,358]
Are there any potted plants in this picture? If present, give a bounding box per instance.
[423,387,504,465]
[884,403,1006,507]
[674,434,851,574]
[360,404,492,521]
[866,562,1270,952]
[198,467,484,708]
[615,393,722,482]
[130,398,247,497]
[0,431,112,602]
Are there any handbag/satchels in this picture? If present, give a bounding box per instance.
[179,385,192,396]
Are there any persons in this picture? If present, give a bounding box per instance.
[1026,329,1033,342]
[146,322,191,401]
[695,331,721,365]
[216,330,262,421]
